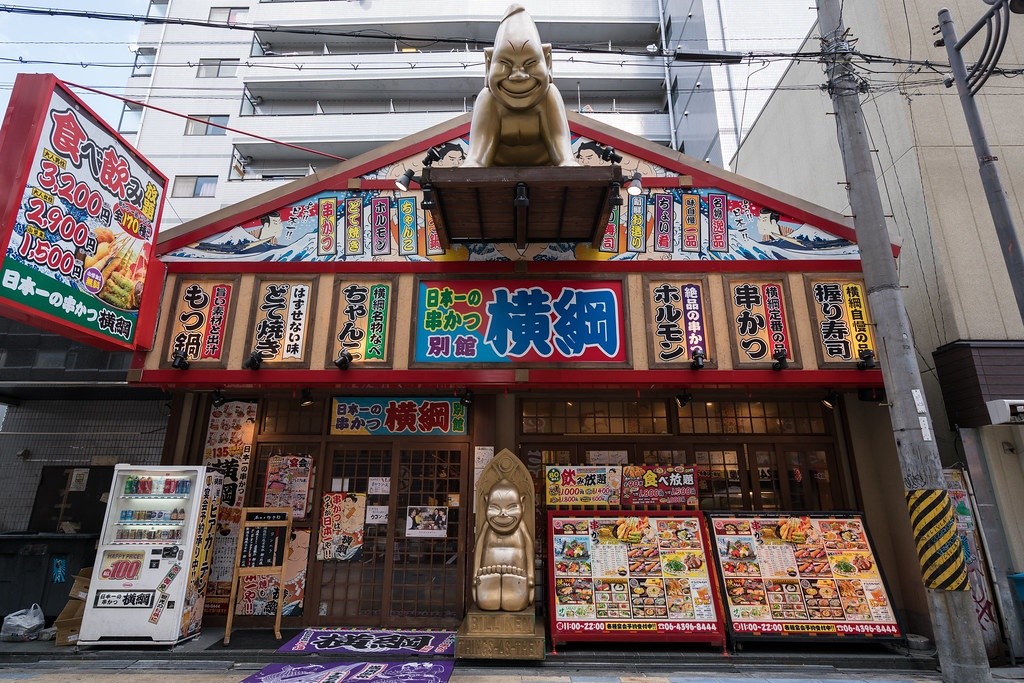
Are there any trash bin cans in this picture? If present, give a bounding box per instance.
[0,530,100,627]
[1007,572,1024,611]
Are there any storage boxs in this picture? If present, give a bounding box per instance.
[68,567,93,601]
[54,616,84,646]
[52,599,85,627]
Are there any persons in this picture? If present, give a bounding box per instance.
[471,480,536,611]
[408,507,447,530]
[465,4,581,166]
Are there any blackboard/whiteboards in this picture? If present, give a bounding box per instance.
[234,506,294,577]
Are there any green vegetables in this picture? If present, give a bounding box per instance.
[835,560,856,572]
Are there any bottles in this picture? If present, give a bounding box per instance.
[124,475,190,495]
[171,507,185,520]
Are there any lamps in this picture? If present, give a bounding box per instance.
[602,145,623,165]
[608,180,623,206]
[300,387,315,406]
[212,388,226,408]
[394,169,421,193]
[857,349,876,370]
[461,390,475,408]
[690,350,705,371]
[334,349,353,369]
[169,349,190,370]
[624,171,643,195]
[241,350,262,370]
[772,351,789,371]
[512,182,530,208]
[421,183,436,210]
[422,146,440,167]
[821,390,837,409]
[675,389,694,409]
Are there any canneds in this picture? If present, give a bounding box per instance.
[120,511,171,521]
[116,529,181,540]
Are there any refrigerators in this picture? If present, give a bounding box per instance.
[75,465,226,646]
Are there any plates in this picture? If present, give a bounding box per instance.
[832,563,858,576]
[90,293,140,312]
[663,562,688,574]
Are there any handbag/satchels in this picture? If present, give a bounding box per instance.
[1,603,44,641]
